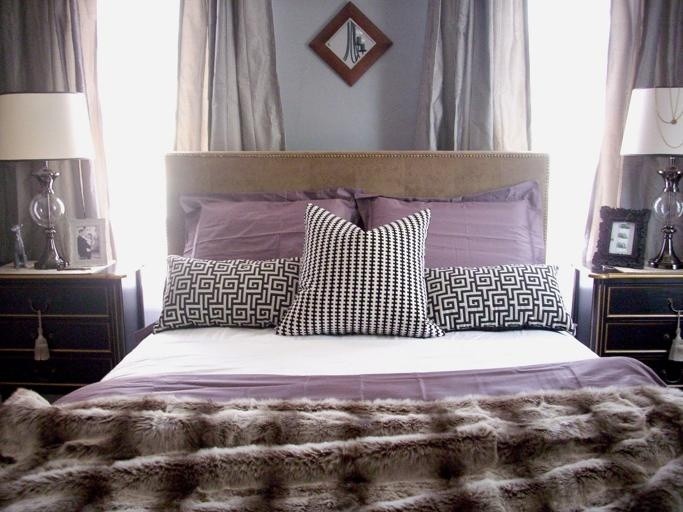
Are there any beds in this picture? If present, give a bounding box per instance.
[0,152,683,512]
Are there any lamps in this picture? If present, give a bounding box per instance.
[620,88,683,270]
[0,93,96,269]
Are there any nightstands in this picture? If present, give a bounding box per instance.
[569,263,683,388]
[0,263,147,403]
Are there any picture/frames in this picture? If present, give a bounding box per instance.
[309,0,393,88]
[592,205,652,269]
[67,218,107,269]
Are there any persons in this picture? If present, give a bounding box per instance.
[85,233,96,259]
[77,229,91,259]
